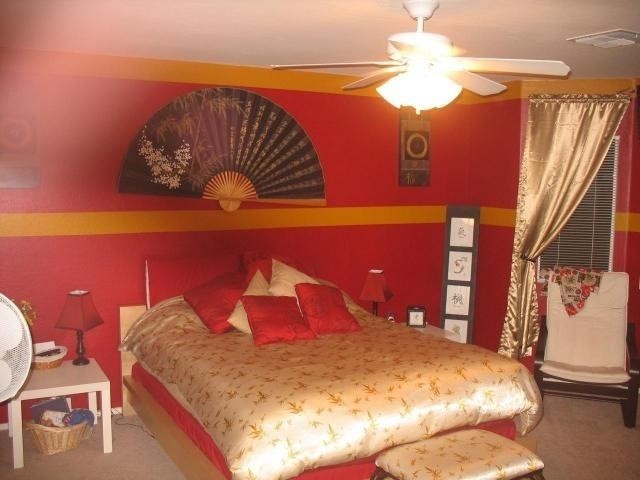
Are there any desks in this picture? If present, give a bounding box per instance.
[6,357,114,467]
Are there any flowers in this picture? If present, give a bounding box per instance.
[19,300,38,327]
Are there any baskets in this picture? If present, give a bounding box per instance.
[24,417,90,457]
[32,345,69,370]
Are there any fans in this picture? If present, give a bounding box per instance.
[271,0,573,97]
[0,292,32,406]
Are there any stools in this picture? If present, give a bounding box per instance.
[370,428,546,480]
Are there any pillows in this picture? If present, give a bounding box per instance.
[181,255,360,343]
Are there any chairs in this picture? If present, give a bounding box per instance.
[535,267,637,429]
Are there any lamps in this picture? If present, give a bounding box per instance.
[358,269,391,315]
[55,289,105,365]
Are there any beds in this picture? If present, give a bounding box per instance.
[119,300,522,479]
